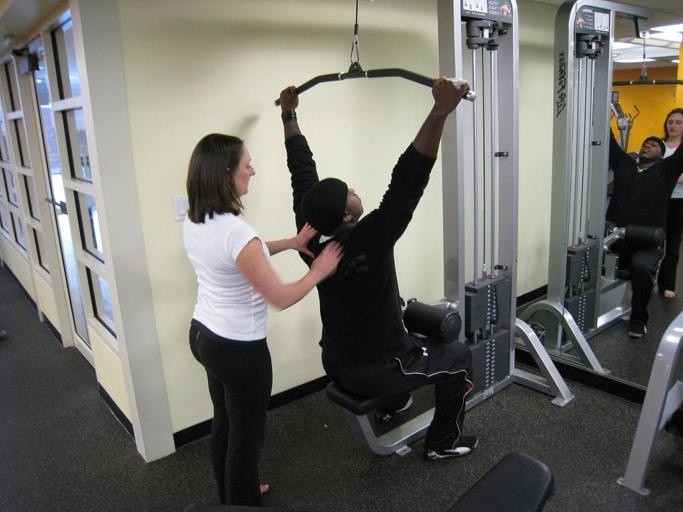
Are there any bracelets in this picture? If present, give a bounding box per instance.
[281,110,297,125]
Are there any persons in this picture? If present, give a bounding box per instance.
[601,126,683,338]
[182,134,346,511]
[280,76,479,462]
[635,108,683,298]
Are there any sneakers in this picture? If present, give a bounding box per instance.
[375,392,413,416]
[628,317,646,338]
[423,434,478,461]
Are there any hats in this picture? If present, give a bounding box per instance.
[644,137,666,155]
[301,178,347,235]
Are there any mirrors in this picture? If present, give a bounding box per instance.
[484,0,683,404]
